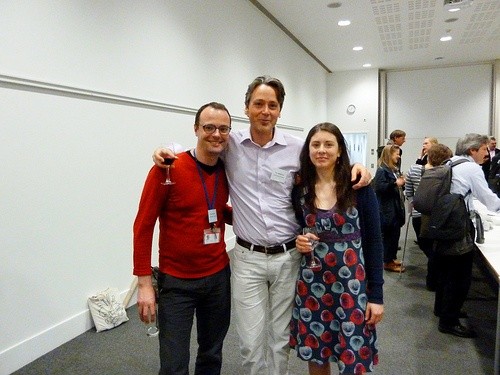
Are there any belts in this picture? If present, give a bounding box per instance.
[236,236,297,255]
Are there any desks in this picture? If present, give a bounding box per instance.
[474,200,500,375]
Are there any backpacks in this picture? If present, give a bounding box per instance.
[412,159,472,213]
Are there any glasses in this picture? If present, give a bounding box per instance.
[202,124,231,133]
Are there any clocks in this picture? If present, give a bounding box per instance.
[348,105,355,113]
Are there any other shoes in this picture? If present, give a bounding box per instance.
[384,261,405,272]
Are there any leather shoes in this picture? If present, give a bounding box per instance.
[433,310,468,319]
[438,323,475,338]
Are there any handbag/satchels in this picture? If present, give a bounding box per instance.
[429,193,468,242]
[152,265,159,302]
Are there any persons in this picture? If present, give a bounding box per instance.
[377,130,406,250]
[479,136,500,199]
[419,143,453,292]
[405,137,438,245]
[434,133,500,338]
[152,75,372,375]
[133,102,233,375]
[375,145,407,272]
[289,123,385,375]
